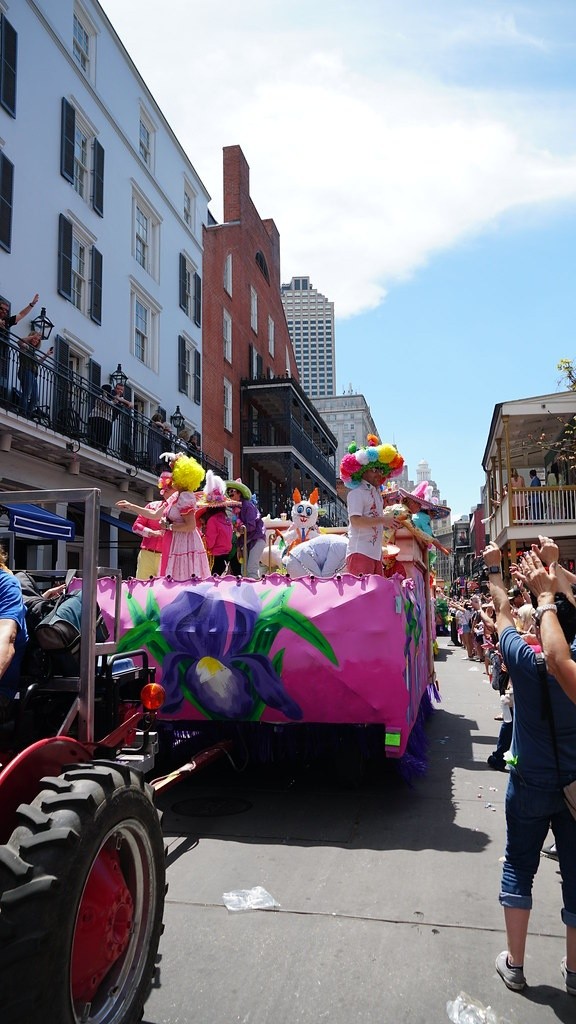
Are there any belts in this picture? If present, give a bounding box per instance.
[145,548,161,553]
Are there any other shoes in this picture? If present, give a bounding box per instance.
[540,843,559,861]
[447,628,517,773]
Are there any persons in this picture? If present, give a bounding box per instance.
[480,469,527,527]
[87,382,134,453]
[287,531,349,577]
[0,542,32,717]
[546,464,567,519]
[113,452,268,579]
[339,434,451,582]
[0,293,40,400]
[17,330,54,418]
[527,469,544,519]
[437,534,576,996]
[145,413,202,476]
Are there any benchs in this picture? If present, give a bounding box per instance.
[96,647,158,755]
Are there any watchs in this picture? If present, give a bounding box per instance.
[486,564,501,575]
[534,604,558,620]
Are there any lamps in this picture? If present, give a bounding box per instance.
[170,405,185,428]
[110,363,128,393]
[30,306,54,341]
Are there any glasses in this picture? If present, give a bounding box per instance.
[369,467,387,475]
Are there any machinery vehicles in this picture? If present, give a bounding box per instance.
[0,485,437,1024]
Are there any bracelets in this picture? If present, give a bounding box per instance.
[29,303,34,307]
[169,524,174,531]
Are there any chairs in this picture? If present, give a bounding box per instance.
[59,407,88,439]
[123,438,149,470]
[12,387,52,429]
[0,607,54,753]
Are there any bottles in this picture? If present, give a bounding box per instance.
[500,695,512,723]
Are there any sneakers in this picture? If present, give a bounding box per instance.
[560,956,576,995]
[494,950,526,989]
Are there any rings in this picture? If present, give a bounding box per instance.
[526,570,532,576]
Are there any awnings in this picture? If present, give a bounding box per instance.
[2,502,75,542]
[99,512,140,542]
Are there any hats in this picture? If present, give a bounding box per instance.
[196,470,242,508]
[398,480,432,510]
[339,433,404,489]
[224,477,252,500]
[430,497,451,520]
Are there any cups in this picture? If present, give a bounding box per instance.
[280,513,287,522]
